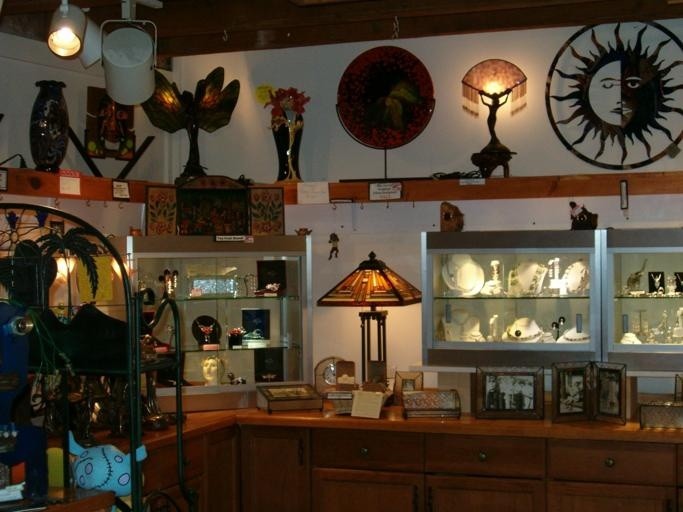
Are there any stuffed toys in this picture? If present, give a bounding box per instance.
[67,429,148,511]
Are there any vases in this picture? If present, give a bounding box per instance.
[267,105,304,185]
[30,79,71,175]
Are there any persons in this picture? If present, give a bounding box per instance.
[562,382,580,406]
[199,355,224,384]
[486,371,534,410]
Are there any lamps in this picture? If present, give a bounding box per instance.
[461,57,529,179]
[0,153,28,171]
[46,1,168,106]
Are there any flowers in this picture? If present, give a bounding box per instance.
[254,83,312,114]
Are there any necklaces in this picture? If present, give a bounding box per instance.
[194,319,217,342]
